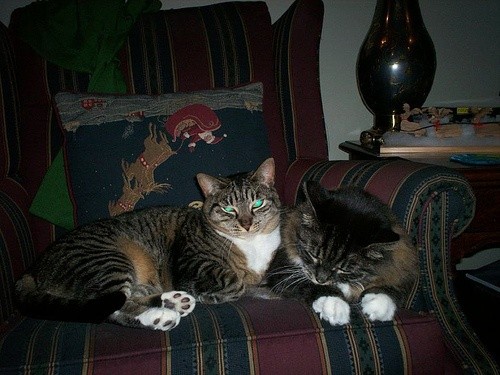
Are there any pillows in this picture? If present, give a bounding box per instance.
[52,79,271,232]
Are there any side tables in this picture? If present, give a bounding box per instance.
[334,105,500,279]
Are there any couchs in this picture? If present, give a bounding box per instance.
[1,1,499,374]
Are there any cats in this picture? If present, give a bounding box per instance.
[265,179,419,325]
[15,157,281,332]
